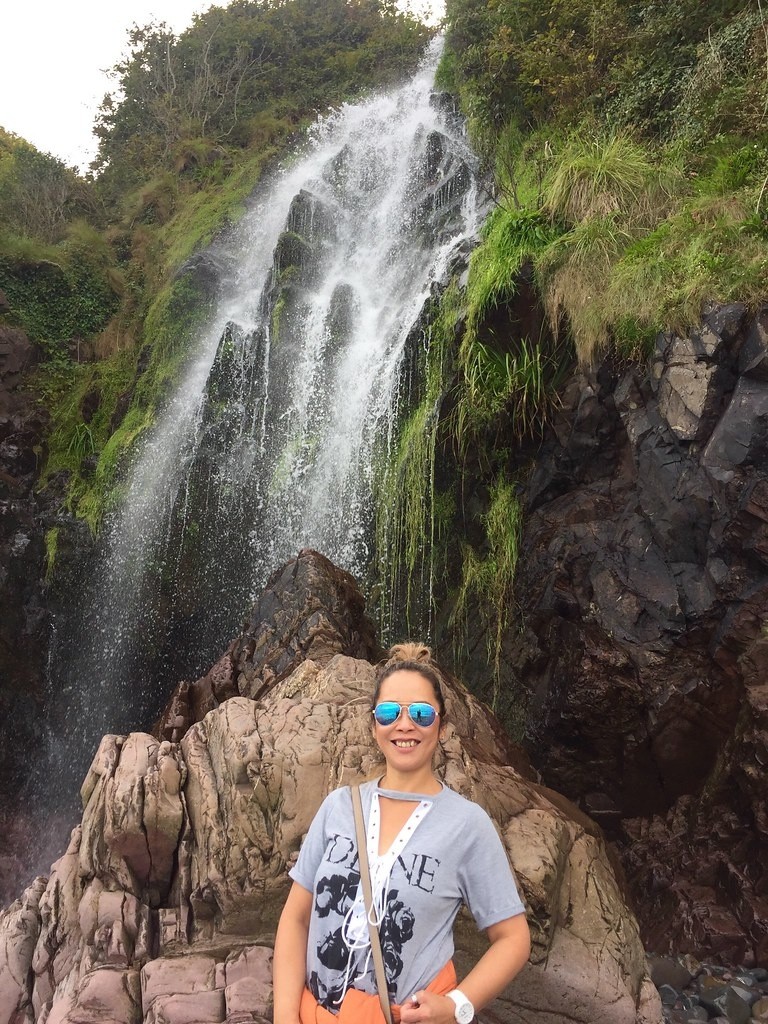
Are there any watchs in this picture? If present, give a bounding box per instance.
[446,989,476,1024]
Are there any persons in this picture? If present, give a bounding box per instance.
[273,643,532,1024]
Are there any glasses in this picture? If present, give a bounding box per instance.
[372,702,442,728]
